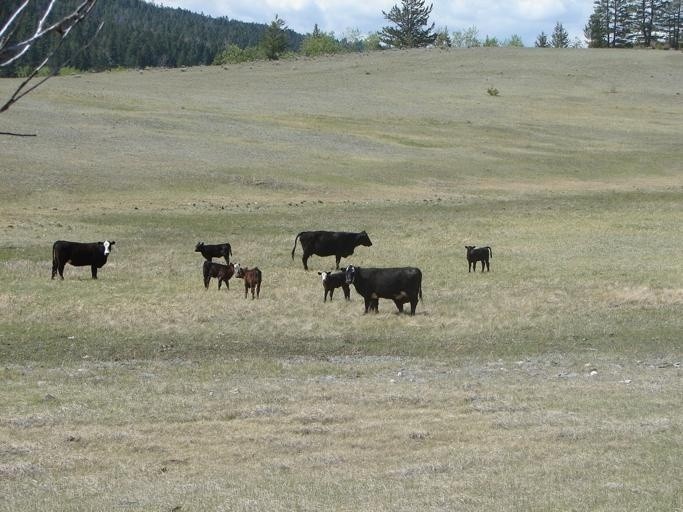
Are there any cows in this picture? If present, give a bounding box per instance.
[292,229,372,270]
[203,261,240,290]
[235,267,262,300]
[194,242,232,265]
[318,271,350,303]
[51,240,116,281]
[369,298,379,314]
[465,246,492,272]
[341,265,424,316]
